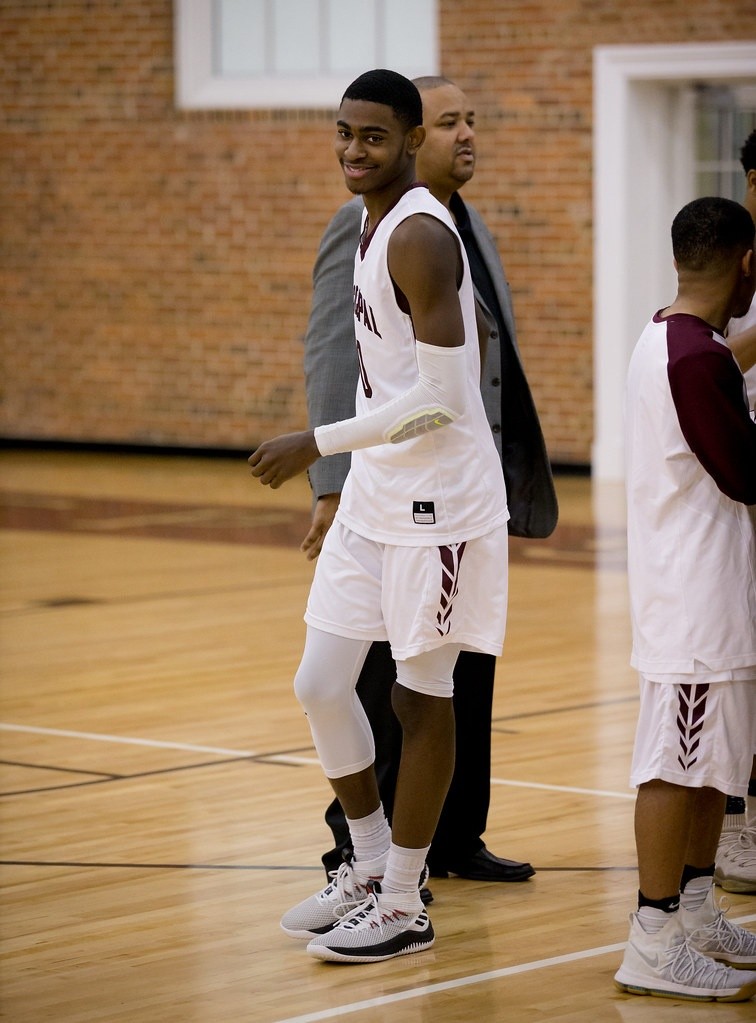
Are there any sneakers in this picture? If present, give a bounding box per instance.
[306,880,435,963]
[279,848,429,939]
[712,813,756,893]
[680,882,756,970]
[613,911,756,1002]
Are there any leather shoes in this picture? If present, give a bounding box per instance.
[429,845,537,882]
[325,868,435,905]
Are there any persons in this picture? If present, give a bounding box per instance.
[246,70,560,963]
[716,124,756,896]
[612,197,756,1002]
